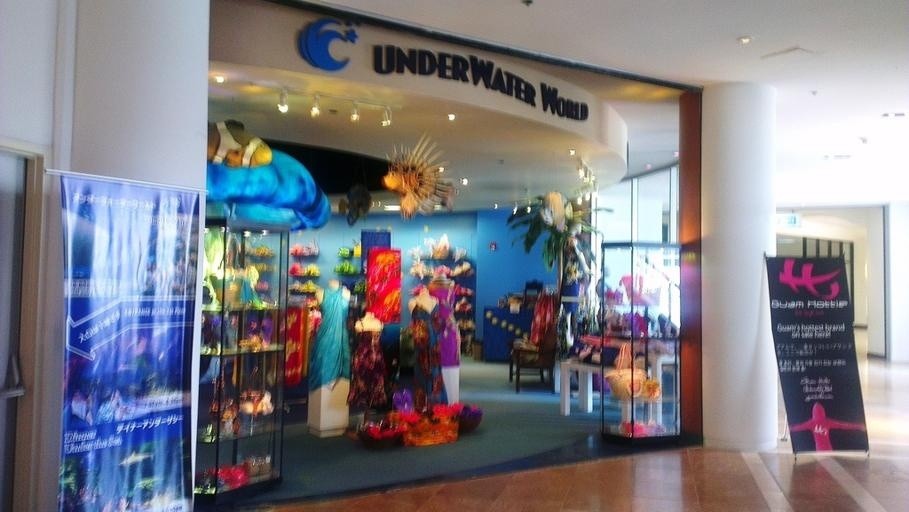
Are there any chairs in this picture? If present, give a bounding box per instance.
[509,296,545,383]
[513,325,554,394]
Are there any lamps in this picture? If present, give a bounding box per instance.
[378,109,391,126]
[310,98,320,117]
[350,104,359,121]
[277,93,288,113]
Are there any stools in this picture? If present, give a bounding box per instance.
[560,356,663,430]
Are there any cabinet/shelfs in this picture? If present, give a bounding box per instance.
[334,249,366,297]
[600,243,680,445]
[288,248,319,298]
[194,215,289,509]
[412,255,473,347]
